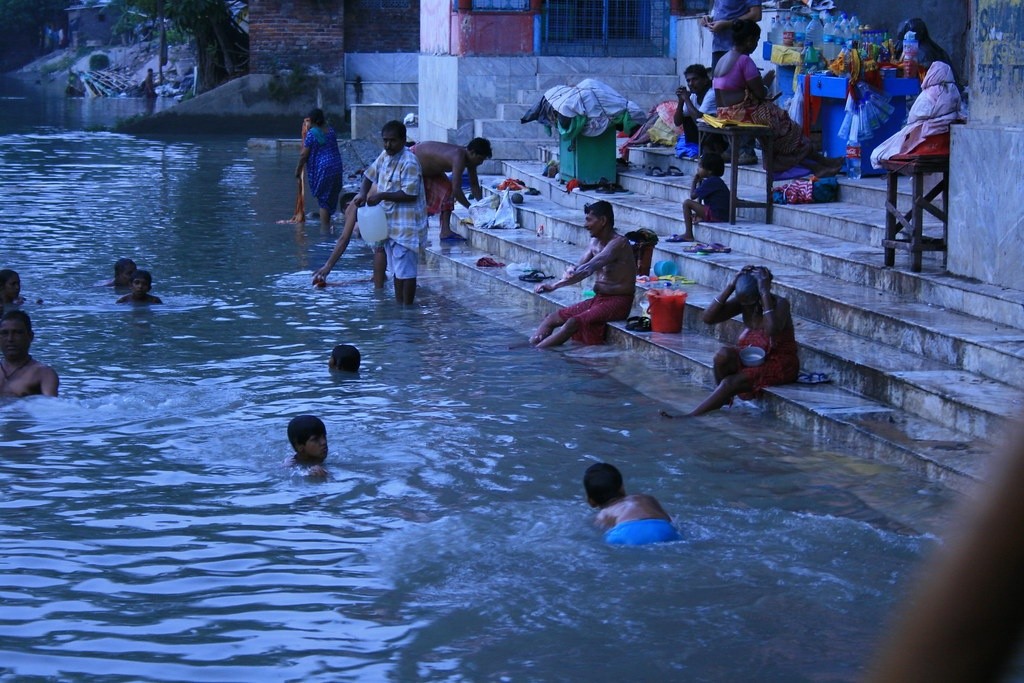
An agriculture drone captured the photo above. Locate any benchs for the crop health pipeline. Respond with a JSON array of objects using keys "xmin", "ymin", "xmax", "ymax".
[
  {"xmin": 697, "ymin": 120, "xmax": 773, "ymax": 224},
  {"xmin": 883, "ymin": 155, "xmax": 950, "ymax": 274}
]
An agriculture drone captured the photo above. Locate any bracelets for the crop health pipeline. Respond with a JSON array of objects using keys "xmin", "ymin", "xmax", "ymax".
[
  {"xmin": 714, "ymin": 298, "xmax": 724, "ymax": 306},
  {"xmin": 763, "ymin": 309, "xmax": 774, "ymax": 316}
]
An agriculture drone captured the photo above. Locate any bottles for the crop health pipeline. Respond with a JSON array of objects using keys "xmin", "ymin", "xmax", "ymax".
[
  {"xmin": 845, "ymin": 138, "xmax": 862, "ymax": 181},
  {"xmin": 838, "ymin": 79, "xmax": 895, "ymax": 141},
  {"xmin": 644, "ymin": 280, "xmax": 674, "ymax": 288},
  {"xmin": 768, "ymin": 7, "xmax": 901, "ymax": 77},
  {"xmin": 902, "ymin": 32, "xmax": 918, "ymax": 77}
]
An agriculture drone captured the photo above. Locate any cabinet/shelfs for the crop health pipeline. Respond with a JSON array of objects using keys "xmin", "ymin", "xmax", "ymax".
[
  {"xmin": 559, "ymin": 126, "xmax": 617, "ymax": 189},
  {"xmin": 796, "ymin": 74, "xmax": 920, "ymax": 175}
]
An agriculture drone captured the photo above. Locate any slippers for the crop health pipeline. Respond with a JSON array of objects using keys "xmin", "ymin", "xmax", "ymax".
[
  {"xmin": 645, "ymin": 166, "xmax": 665, "ymax": 177},
  {"xmin": 625, "ymin": 316, "xmax": 651, "ymax": 331},
  {"xmin": 519, "ymin": 271, "xmax": 555, "ymax": 282},
  {"xmin": 665, "ymin": 234, "xmax": 688, "ymax": 241},
  {"xmin": 683, "ymin": 243, "xmax": 713, "ymax": 252},
  {"xmin": 797, "ymin": 372, "xmax": 830, "ymax": 384},
  {"xmin": 441, "ymin": 234, "xmax": 467, "ymax": 241},
  {"xmin": 664, "ymin": 166, "xmax": 684, "ymax": 175},
  {"xmin": 596, "ymin": 183, "xmax": 629, "ymax": 193},
  {"xmin": 709, "ymin": 242, "xmax": 731, "ymax": 253}
]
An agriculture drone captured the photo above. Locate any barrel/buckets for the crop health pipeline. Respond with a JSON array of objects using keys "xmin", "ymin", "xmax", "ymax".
[
  {"xmin": 654, "ymin": 260, "xmax": 681, "ymax": 278},
  {"xmin": 357, "ymin": 200, "xmax": 389, "ymax": 243},
  {"xmin": 646, "ymin": 289, "xmax": 687, "ymax": 334}
]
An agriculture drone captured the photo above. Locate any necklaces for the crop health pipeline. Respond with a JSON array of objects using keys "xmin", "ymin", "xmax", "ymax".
[{"xmin": 0, "ymin": 354, "xmax": 32, "ymax": 383}]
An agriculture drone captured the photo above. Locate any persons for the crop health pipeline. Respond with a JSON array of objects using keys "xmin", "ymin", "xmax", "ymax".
[
  {"xmin": 145, "ymin": 68, "xmax": 158, "ymax": 98},
  {"xmin": 102, "ymin": 258, "xmax": 163, "ymax": 304},
  {"xmin": 659, "ymin": 264, "xmax": 800, "ymax": 419},
  {"xmin": 0, "ymin": 269, "xmax": 24, "ymax": 315},
  {"xmin": 583, "ymin": 463, "xmax": 679, "ymax": 545},
  {"xmin": 674, "ymin": 64, "xmax": 728, "ymax": 160},
  {"xmin": 281, "ymin": 415, "xmax": 329, "ymax": 479},
  {"xmin": 865, "ymin": 412, "xmax": 1024, "ymax": 683},
  {"xmin": 295, "ymin": 108, "xmax": 344, "ymax": 226},
  {"xmin": 329, "ymin": 345, "xmax": 361, "ymax": 382},
  {"xmin": 700, "ymin": 0, "xmax": 762, "ymax": 79},
  {"xmin": 896, "ymin": 17, "xmax": 964, "ymax": 93},
  {"xmin": 354, "ymin": 77, "xmax": 364, "ymax": 104},
  {"xmin": 312, "ymin": 192, "xmax": 386, "ymax": 287},
  {"xmin": 528, "ymin": 200, "xmax": 638, "ymax": 349},
  {"xmin": 408, "ymin": 138, "xmax": 492, "ymax": 239},
  {"xmin": 354, "ymin": 119, "xmax": 428, "ymax": 305},
  {"xmin": 672, "ymin": 153, "xmax": 731, "ymax": 241},
  {"xmin": 0, "ymin": 310, "xmax": 59, "ymax": 399},
  {"xmin": 713, "ymin": 19, "xmax": 845, "ymax": 177}
]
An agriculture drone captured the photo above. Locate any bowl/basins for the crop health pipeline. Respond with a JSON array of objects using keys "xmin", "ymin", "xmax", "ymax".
[{"xmin": 740, "ymin": 346, "xmax": 766, "ymax": 365}]
